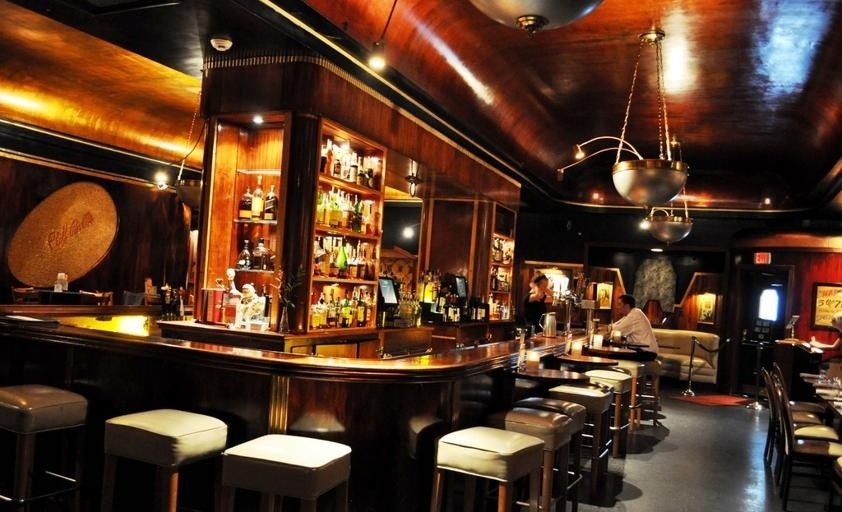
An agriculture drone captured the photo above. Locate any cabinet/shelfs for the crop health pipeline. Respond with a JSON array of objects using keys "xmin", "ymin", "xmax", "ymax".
[
  {"xmin": 200, "ymin": 113, "xmax": 384, "ymax": 332},
  {"xmin": 290, "ymin": 338, "xmax": 379, "ymax": 359},
  {"xmin": 488, "ymin": 232, "xmax": 514, "ymax": 320}
]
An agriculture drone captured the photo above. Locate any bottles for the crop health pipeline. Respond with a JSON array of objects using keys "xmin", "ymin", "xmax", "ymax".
[
  {"xmin": 310, "ymin": 135, "xmax": 377, "ymax": 328},
  {"xmin": 55, "ymin": 273, "xmax": 68, "ymax": 290},
  {"xmin": 490, "ymin": 237, "xmax": 512, "ymax": 319},
  {"xmin": 393, "ymin": 281, "xmax": 421, "ymax": 326},
  {"xmin": 201, "ymin": 174, "xmax": 277, "ymax": 334},
  {"xmin": 159, "ymin": 284, "xmax": 185, "ymax": 321},
  {"xmin": 422, "ymin": 268, "xmax": 489, "ymax": 323}
]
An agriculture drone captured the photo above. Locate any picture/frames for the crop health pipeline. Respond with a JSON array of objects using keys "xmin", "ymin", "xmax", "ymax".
[
  {"xmin": 696, "ymin": 274, "xmax": 718, "ymax": 325},
  {"xmin": 808, "ymin": 282, "xmax": 842, "ymax": 331},
  {"xmin": 594, "ymin": 269, "xmax": 616, "ymax": 311}
]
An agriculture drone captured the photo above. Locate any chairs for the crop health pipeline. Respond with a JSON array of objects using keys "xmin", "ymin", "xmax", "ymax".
[{"xmin": 760, "ymin": 361, "xmax": 842, "ymax": 512}]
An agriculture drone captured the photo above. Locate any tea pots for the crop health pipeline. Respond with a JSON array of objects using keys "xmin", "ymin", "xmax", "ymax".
[{"xmin": 538, "ymin": 311, "xmax": 559, "ymax": 338}]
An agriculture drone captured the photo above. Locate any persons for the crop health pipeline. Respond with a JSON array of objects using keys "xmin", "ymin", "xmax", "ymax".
[
  {"xmin": 522, "ymin": 273, "xmax": 555, "ymax": 334},
  {"xmin": 600, "ymin": 295, "xmax": 659, "ymax": 364},
  {"xmin": 809, "ymin": 312, "xmax": 842, "ymax": 377}
]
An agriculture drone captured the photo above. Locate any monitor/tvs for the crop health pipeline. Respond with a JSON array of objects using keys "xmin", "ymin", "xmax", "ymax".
[{"xmin": 378, "ymin": 277, "xmax": 399, "ymax": 305}]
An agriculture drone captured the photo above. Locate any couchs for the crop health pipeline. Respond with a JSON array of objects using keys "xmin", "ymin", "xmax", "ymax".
[{"xmin": 652, "ymin": 328, "xmax": 721, "ymax": 384}]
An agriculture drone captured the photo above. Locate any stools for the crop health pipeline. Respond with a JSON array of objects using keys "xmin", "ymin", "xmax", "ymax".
[
  {"xmin": 428, "ymin": 358, "xmax": 663, "ymax": 512},
  {"xmin": 100, "ymin": 408, "xmax": 228, "ymax": 512},
  {"xmin": 217, "ymin": 433, "xmax": 353, "ymax": 512},
  {"xmin": 0, "ymin": 385, "xmax": 89, "ymax": 512}
]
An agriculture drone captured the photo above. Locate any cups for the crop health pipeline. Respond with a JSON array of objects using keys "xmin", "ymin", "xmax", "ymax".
[
  {"xmin": 559, "ymin": 298, "xmax": 572, "ymax": 336},
  {"xmin": 594, "ymin": 335, "xmax": 604, "ymax": 348},
  {"xmin": 514, "ymin": 326, "xmax": 535, "ymax": 340},
  {"xmin": 526, "ymin": 351, "xmax": 540, "ymax": 373},
  {"xmin": 54, "ymin": 284, "xmax": 62, "ymax": 292},
  {"xmin": 584, "ymin": 318, "xmax": 601, "ymax": 334},
  {"xmin": 571, "ymin": 342, "xmax": 583, "ymax": 358},
  {"xmin": 614, "ymin": 331, "xmax": 622, "ymax": 343},
  {"xmin": 378, "ymin": 311, "xmax": 386, "ymax": 328}
]
